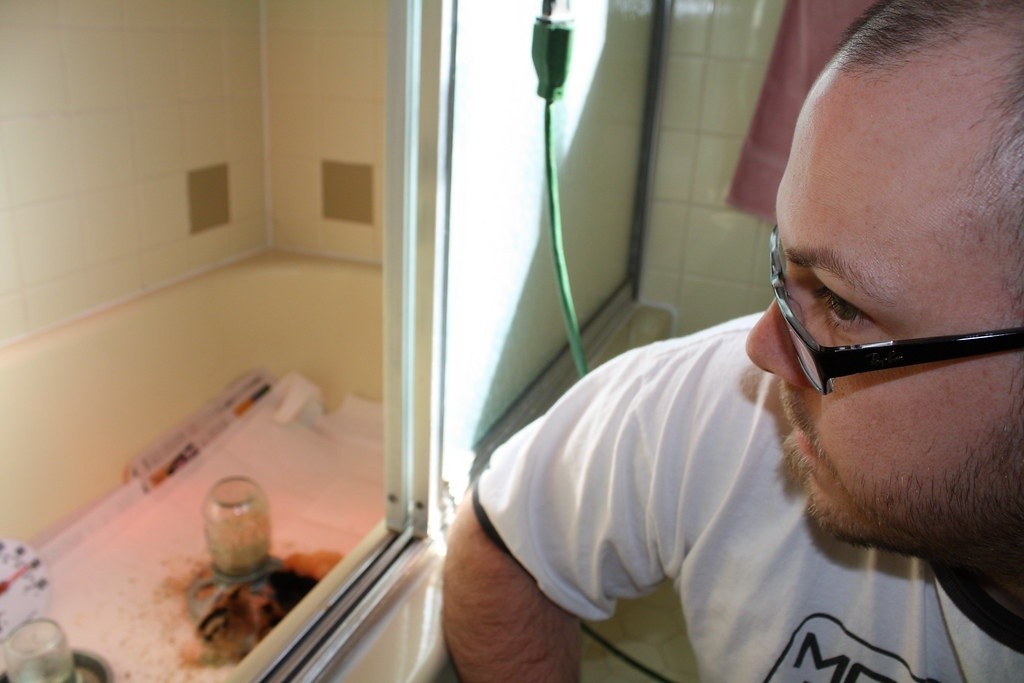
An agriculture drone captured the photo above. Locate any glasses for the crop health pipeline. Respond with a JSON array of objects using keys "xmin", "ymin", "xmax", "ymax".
[{"xmin": 769, "ymin": 225, "xmax": 1023, "ymax": 396}]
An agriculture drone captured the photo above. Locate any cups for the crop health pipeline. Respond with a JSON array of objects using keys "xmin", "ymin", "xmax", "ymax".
[
  {"xmin": 203, "ymin": 475, "xmax": 272, "ymax": 579},
  {"xmin": 4, "ymin": 618, "xmax": 83, "ymax": 682}
]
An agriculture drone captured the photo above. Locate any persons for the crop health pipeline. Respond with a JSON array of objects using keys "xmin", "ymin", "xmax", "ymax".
[{"xmin": 442, "ymin": 0, "xmax": 1024, "ymax": 683}]
